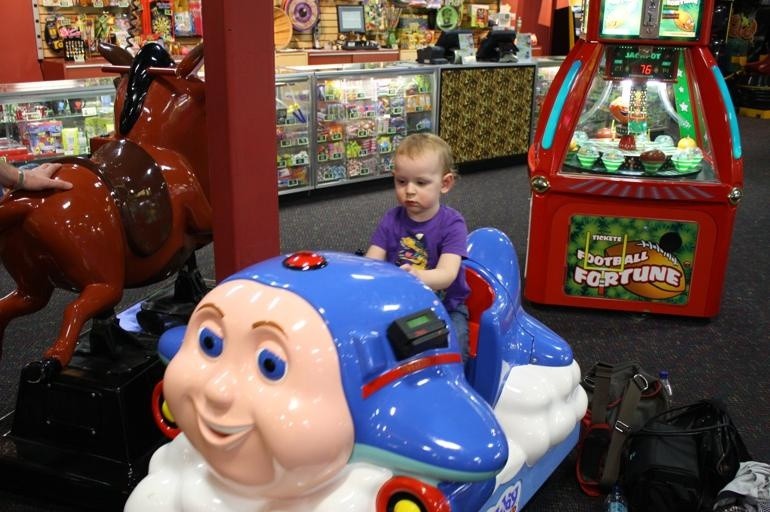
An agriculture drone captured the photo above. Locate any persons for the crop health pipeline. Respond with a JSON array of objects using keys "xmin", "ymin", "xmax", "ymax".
[
  {"xmin": 361, "ymin": 134, "xmax": 468, "ymax": 368},
  {"xmin": 1, "ymin": 157, "xmax": 74, "ymax": 193}
]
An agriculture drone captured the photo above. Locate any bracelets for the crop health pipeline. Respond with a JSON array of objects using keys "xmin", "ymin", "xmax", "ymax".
[{"xmin": 9, "ymin": 169, "xmax": 24, "ymax": 194}]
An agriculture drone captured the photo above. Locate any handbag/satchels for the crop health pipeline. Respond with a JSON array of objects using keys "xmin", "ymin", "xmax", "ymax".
[{"xmin": 567, "ymin": 362, "xmax": 770, "ymax": 512}]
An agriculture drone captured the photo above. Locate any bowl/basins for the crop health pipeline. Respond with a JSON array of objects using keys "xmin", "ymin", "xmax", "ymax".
[{"xmin": 570, "ymin": 133, "xmax": 706, "ymax": 174}]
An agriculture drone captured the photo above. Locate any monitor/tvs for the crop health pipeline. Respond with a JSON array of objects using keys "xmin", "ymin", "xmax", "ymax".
[
  {"xmin": 337, "ymin": 4, "xmax": 366, "ymax": 34},
  {"xmin": 599, "ymin": 0, "xmax": 643, "ymax": 39},
  {"xmin": 659, "ymin": 0, "xmax": 705, "ymax": 41},
  {"xmin": 437, "ymin": 31, "xmax": 474, "ymax": 64},
  {"xmin": 476, "ymin": 30, "xmax": 519, "ymax": 62}
]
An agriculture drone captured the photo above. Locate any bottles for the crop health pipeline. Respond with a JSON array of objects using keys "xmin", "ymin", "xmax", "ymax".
[{"xmin": 656, "ymin": 371, "xmax": 674, "ymax": 416}]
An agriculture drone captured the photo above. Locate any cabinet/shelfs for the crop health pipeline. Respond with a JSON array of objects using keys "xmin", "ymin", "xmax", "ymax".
[{"xmin": 0, "ymin": 75, "xmax": 119, "ymax": 203}]
